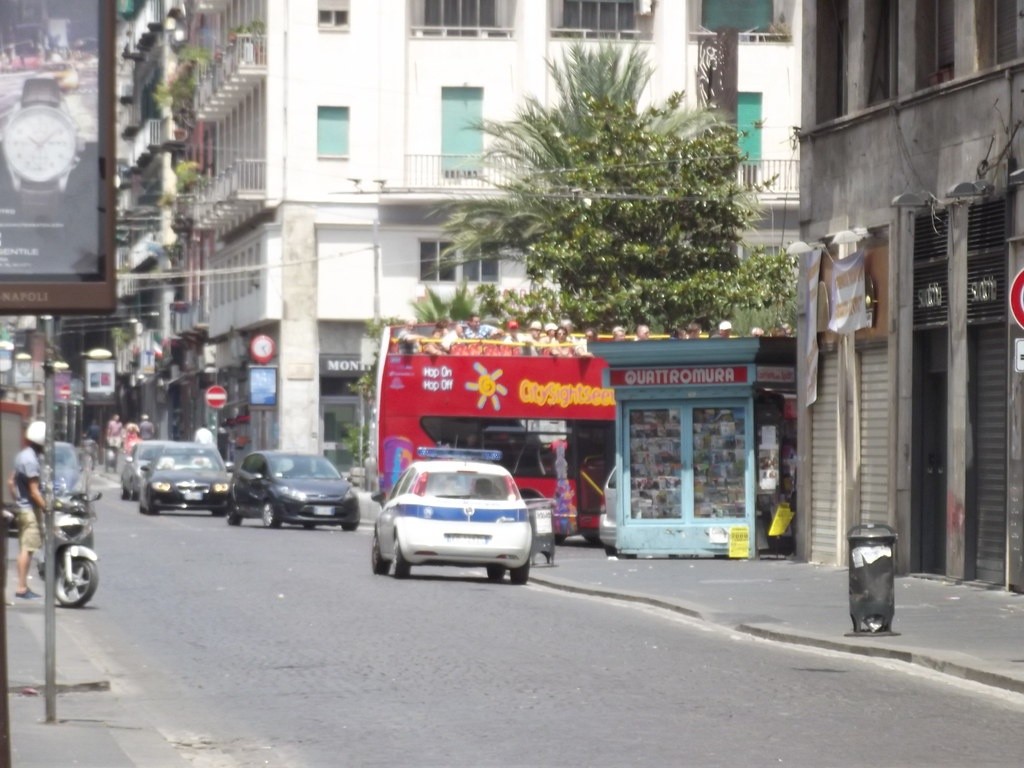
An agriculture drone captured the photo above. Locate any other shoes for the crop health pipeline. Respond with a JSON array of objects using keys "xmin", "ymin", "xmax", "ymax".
[{"xmin": 17, "ymin": 586, "xmax": 44, "ymax": 602}]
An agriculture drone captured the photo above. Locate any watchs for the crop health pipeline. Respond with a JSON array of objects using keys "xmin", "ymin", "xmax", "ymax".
[{"xmin": 2, "ymin": 79, "xmax": 82, "ymax": 222}]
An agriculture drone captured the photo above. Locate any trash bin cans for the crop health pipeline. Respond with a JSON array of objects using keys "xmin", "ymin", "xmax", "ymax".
[
  {"xmin": 843, "ymin": 523, "xmax": 903, "ymax": 637},
  {"xmin": 522, "ymin": 498, "xmax": 559, "ymax": 569}
]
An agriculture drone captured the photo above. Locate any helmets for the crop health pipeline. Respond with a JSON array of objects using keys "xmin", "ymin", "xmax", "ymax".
[{"xmin": 26, "ymin": 419, "xmax": 47, "ymax": 446}]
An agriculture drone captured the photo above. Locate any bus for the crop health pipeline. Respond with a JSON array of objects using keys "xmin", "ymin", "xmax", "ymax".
[{"xmin": 375, "ymin": 321, "xmax": 615, "ymax": 543}]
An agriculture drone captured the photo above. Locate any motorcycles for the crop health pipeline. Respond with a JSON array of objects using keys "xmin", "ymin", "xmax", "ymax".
[{"xmin": 38, "ymin": 482, "xmax": 100, "ymax": 606}]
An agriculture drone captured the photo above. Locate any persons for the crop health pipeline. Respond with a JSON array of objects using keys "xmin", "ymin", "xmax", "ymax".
[
  {"xmin": 82, "ymin": 418, "xmax": 100, "ymax": 443},
  {"xmin": 194, "ymin": 423, "xmax": 212, "ymax": 444},
  {"xmin": 6, "ymin": 419, "xmax": 53, "ymax": 598},
  {"xmin": 137, "ymin": 414, "xmax": 155, "ymax": 440},
  {"xmin": 106, "ymin": 414, "xmax": 123, "ymax": 455},
  {"xmin": 217, "ymin": 421, "xmax": 232, "ymax": 461},
  {"xmin": 122, "ymin": 422, "xmax": 140, "ymax": 456}
]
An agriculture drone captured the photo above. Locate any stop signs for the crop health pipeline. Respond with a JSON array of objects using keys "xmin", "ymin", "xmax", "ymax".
[{"xmin": 206, "ymin": 385, "xmax": 227, "ymax": 408}]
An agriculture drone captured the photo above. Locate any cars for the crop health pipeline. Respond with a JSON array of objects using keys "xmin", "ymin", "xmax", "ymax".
[
  {"xmin": 372, "ymin": 447, "xmax": 533, "ymax": 584},
  {"xmin": 227, "ymin": 451, "xmax": 360, "ymax": 531},
  {"xmin": 122, "ymin": 441, "xmax": 166, "ymax": 501},
  {"xmin": 141, "ymin": 442, "xmax": 234, "ymax": 517},
  {"xmin": 599, "ymin": 465, "xmax": 616, "ymax": 556},
  {"xmin": 53, "ymin": 442, "xmax": 86, "ymax": 493}
]
